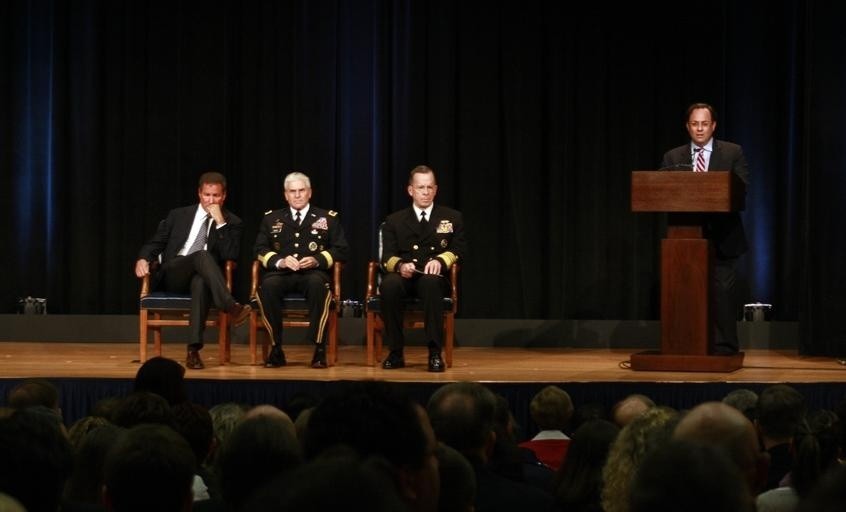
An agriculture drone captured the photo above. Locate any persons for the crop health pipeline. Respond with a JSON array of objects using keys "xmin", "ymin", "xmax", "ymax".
[
  {"xmin": 659, "ymin": 101, "xmax": 749, "ymax": 354},
  {"xmin": 378, "ymin": 165, "xmax": 469, "ymax": 368},
  {"xmin": 134, "ymin": 172, "xmax": 251, "ymax": 369},
  {"xmin": 1, "ymin": 356, "xmax": 846, "ymax": 511},
  {"xmin": 252, "ymin": 171, "xmax": 349, "ymax": 369}
]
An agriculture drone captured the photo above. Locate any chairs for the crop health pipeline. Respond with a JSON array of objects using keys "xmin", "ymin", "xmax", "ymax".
[
  {"xmin": 136, "ymin": 217, "xmax": 238, "ymax": 367},
  {"xmin": 248, "ymin": 251, "xmax": 343, "ymax": 368},
  {"xmin": 364, "ymin": 221, "xmax": 457, "ymax": 368}
]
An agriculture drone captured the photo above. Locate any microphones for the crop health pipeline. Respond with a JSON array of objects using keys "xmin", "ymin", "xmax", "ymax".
[{"xmin": 693, "ymin": 147, "xmax": 701, "ymax": 152}]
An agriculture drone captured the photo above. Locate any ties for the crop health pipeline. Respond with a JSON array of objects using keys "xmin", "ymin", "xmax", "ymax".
[
  {"xmin": 420, "ymin": 210, "xmax": 427, "ymax": 227},
  {"xmin": 295, "ymin": 212, "xmax": 301, "ymax": 225},
  {"xmin": 187, "ymin": 215, "xmax": 212, "ymax": 255},
  {"xmin": 696, "ymin": 147, "xmax": 705, "ymax": 172}
]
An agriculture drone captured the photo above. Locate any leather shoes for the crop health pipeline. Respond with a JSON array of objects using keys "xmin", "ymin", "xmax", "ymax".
[
  {"xmin": 429, "ymin": 350, "xmax": 444, "ymax": 372},
  {"xmin": 265, "ymin": 349, "xmax": 287, "ymax": 367},
  {"xmin": 186, "ymin": 349, "xmax": 204, "ymax": 369},
  {"xmin": 383, "ymin": 352, "xmax": 404, "ymax": 369},
  {"xmin": 229, "ymin": 301, "xmax": 251, "ymax": 329},
  {"xmin": 311, "ymin": 349, "xmax": 328, "ymax": 368}
]
